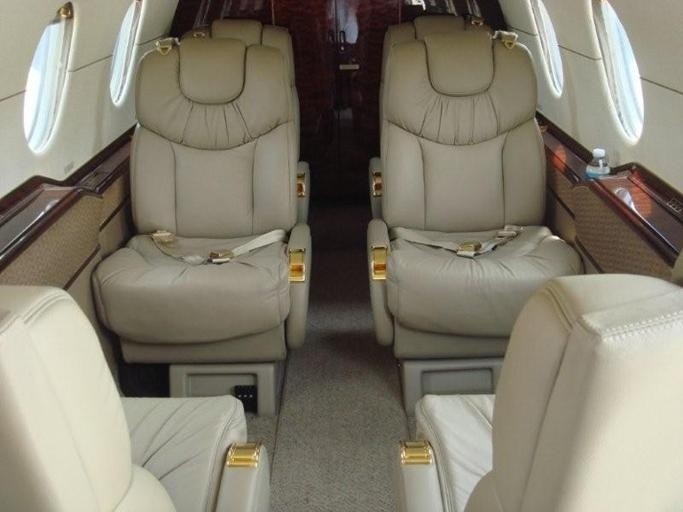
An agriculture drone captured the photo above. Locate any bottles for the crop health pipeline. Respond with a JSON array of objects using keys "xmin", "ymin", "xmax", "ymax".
[{"xmin": 585, "ymin": 148, "xmax": 611, "ymax": 182}]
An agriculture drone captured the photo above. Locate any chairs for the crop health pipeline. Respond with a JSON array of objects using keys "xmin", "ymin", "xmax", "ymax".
[
  {"xmin": 183, "ymin": 20, "xmax": 311, "ymax": 221},
  {"xmin": 400, "ymin": 268, "xmax": 683, "ymax": 510},
  {"xmin": 368, "ymin": 16, "xmax": 489, "ymax": 222},
  {"xmin": 0, "ymin": 280, "xmax": 273, "ymax": 512},
  {"xmin": 92, "ymin": 37, "xmax": 312, "ymax": 367},
  {"xmin": 367, "ymin": 30, "xmax": 586, "ymax": 361}
]
